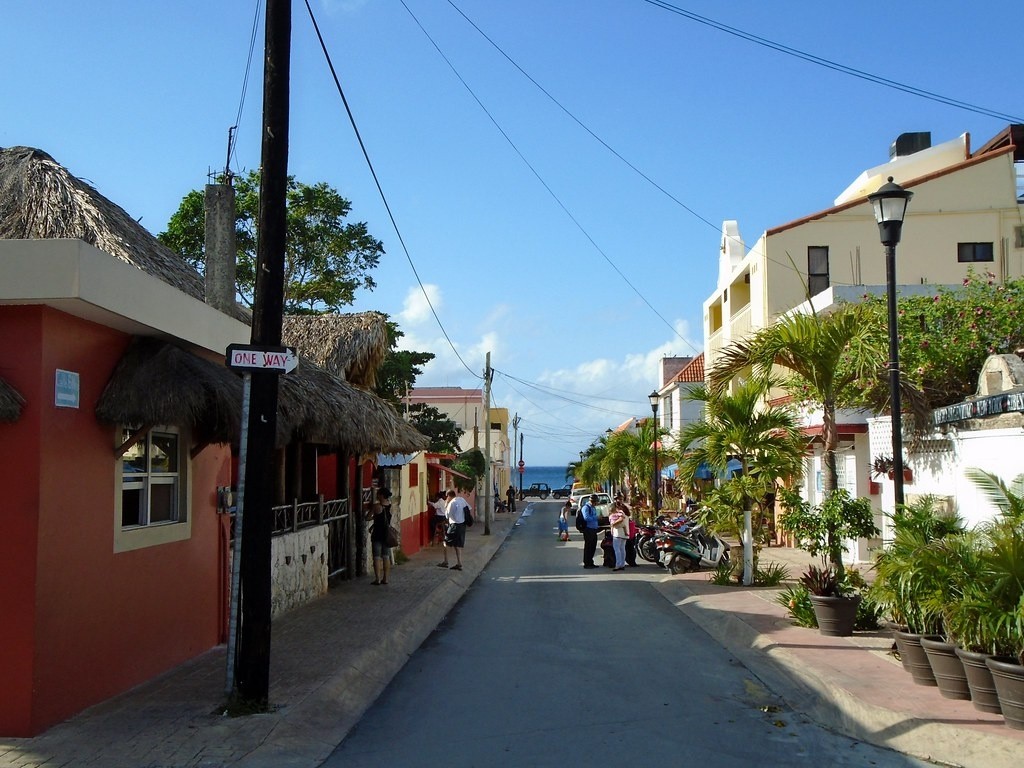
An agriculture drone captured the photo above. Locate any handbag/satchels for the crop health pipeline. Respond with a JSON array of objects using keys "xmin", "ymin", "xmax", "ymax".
[
  {"xmin": 385, "ymin": 524, "xmax": 399, "ymax": 548},
  {"xmin": 459, "ymin": 497, "xmax": 475, "ymax": 527}
]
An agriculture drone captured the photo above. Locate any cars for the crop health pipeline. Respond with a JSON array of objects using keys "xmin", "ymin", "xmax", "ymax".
[
  {"xmin": 576, "ymin": 492, "xmax": 614, "ymax": 526},
  {"xmin": 551, "ymin": 484, "xmax": 572, "ymax": 499},
  {"xmin": 569, "ymin": 488, "xmax": 592, "ymax": 516}
]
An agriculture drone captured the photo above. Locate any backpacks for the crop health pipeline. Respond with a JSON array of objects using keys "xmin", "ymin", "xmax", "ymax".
[{"xmin": 576, "ymin": 504, "xmax": 591, "ymax": 534}]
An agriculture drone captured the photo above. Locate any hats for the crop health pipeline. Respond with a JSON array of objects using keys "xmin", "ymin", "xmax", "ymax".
[
  {"xmin": 588, "ymin": 494, "xmax": 599, "ymax": 501},
  {"xmin": 614, "ymin": 491, "xmax": 625, "ymax": 500}
]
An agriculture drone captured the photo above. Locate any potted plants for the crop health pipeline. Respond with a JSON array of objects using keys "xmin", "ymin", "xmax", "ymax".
[
  {"xmin": 778, "ymin": 484, "xmax": 882, "ymax": 637},
  {"xmin": 689, "ymin": 470, "xmax": 776, "ymax": 579},
  {"xmin": 867, "ymin": 468, "xmax": 1024, "ymax": 730}
]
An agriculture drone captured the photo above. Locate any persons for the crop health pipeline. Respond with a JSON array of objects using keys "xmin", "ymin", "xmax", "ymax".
[
  {"xmin": 494, "ymin": 483, "xmax": 519, "ymax": 514},
  {"xmin": 366, "ymin": 488, "xmax": 392, "ymax": 586},
  {"xmin": 557, "ymin": 483, "xmax": 771, "ymax": 572},
  {"xmin": 427, "ymin": 489, "xmax": 472, "ymax": 571}
]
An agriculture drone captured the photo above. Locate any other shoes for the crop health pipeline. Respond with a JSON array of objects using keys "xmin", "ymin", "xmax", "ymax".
[
  {"xmin": 583, "ymin": 564, "xmax": 599, "ymax": 570},
  {"xmin": 438, "ymin": 561, "xmax": 449, "ymax": 567},
  {"xmin": 381, "ymin": 579, "xmax": 389, "ymax": 584},
  {"xmin": 450, "ymin": 564, "xmax": 463, "ymax": 571},
  {"xmin": 613, "ymin": 567, "xmax": 625, "ymax": 571},
  {"xmin": 370, "ymin": 580, "xmax": 381, "ymax": 585}
]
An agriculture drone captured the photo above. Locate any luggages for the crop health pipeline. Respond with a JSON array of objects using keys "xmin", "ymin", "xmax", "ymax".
[{"xmin": 601, "ymin": 527, "xmax": 617, "ymax": 569}]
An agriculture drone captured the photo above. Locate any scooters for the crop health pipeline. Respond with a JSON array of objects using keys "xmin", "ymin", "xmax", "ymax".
[{"xmin": 632, "ymin": 502, "xmax": 734, "ymax": 576}]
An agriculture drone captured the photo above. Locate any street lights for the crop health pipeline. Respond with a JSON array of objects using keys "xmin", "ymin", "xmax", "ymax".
[
  {"xmin": 578, "ymin": 450, "xmax": 585, "ymax": 463},
  {"xmin": 866, "ymin": 175, "xmax": 916, "ymax": 536},
  {"xmin": 648, "ymin": 390, "xmax": 662, "ymax": 519},
  {"xmin": 605, "ymin": 428, "xmax": 614, "ymax": 495}
]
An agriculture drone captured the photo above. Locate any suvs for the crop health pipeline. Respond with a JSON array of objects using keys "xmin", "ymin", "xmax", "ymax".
[{"xmin": 518, "ymin": 482, "xmax": 551, "ymax": 501}]
